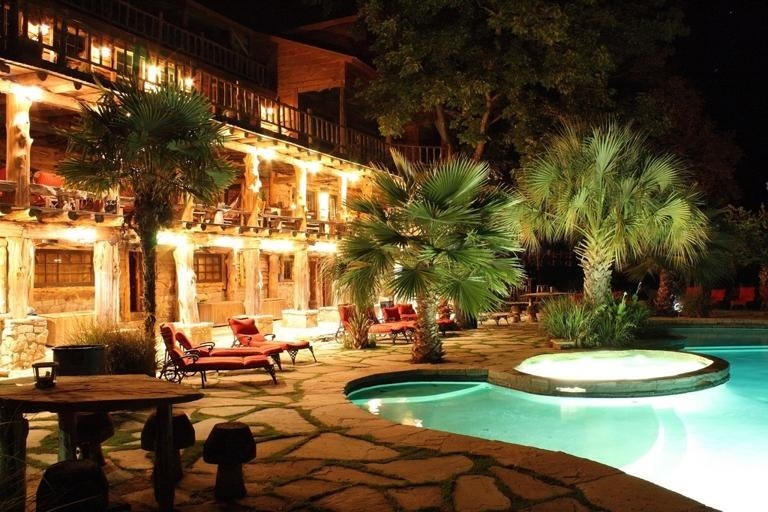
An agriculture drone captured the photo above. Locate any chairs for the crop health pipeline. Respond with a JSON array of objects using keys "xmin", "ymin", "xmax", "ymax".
[
  {"xmin": 571, "ymin": 286, "xmax": 758, "ymax": 312},
  {"xmin": 333, "ymin": 294, "xmax": 461, "ymax": 345},
  {"xmin": 154, "ymin": 322, "xmax": 282, "ymax": 391},
  {"xmin": 171, "ymin": 326, "xmax": 279, "ymax": 385},
  {"xmin": 225, "ymin": 315, "xmax": 319, "ymax": 371}
]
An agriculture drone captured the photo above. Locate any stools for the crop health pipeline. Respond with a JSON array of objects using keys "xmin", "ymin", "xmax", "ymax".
[
  {"xmin": 58, "ymin": 414, "xmax": 115, "ymax": 468},
  {"xmin": 199, "ymin": 421, "xmax": 258, "ymax": 504},
  {"xmin": 136, "ymin": 410, "xmax": 200, "ymax": 487},
  {"xmin": 33, "ymin": 458, "xmax": 113, "ymax": 512}
]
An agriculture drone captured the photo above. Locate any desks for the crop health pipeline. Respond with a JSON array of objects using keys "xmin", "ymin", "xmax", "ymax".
[
  {"xmin": 1, "ymin": 372, "xmax": 208, "ymax": 511},
  {"xmin": 482, "ymin": 289, "xmax": 570, "ymax": 327}
]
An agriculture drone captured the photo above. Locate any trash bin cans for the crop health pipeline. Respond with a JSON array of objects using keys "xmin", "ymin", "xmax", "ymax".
[{"xmin": 52, "ymin": 344, "xmax": 109, "ymax": 431}]
